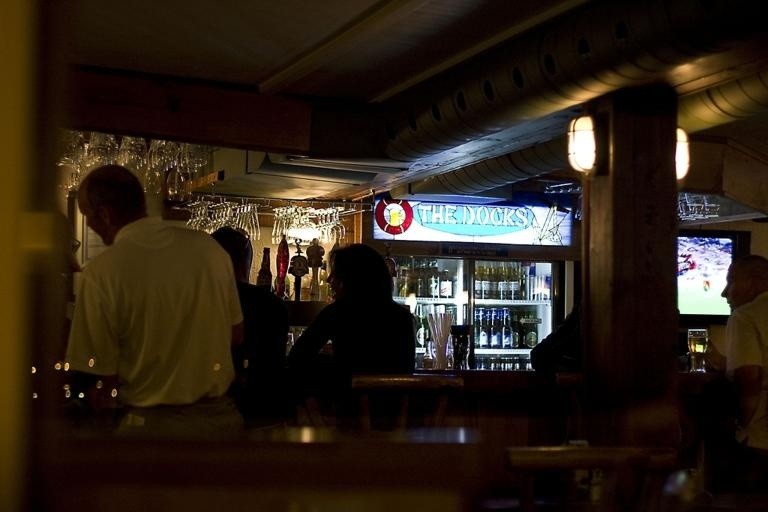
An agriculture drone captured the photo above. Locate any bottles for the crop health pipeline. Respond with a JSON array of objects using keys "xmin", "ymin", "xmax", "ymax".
[
  {"xmin": 256, "ymin": 248, "xmax": 272, "ymax": 295},
  {"xmin": 394, "ymin": 262, "xmax": 536, "ymax": 372}
]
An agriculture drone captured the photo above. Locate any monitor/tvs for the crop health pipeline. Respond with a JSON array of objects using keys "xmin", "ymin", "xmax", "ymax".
[{"xmin": 673, "ymin": 228, "xmax": 751, "ymax": 324}]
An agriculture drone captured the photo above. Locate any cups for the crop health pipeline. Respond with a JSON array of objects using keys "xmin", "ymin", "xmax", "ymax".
[{"xmin": 686, "ymin": 326, "xmax": 708, "ymax": 374}]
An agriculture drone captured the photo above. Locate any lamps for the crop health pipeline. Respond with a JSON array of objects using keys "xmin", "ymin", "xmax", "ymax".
[{"xmin": 565, "ymin": 110, "xmax": 609, "ymax": 178}]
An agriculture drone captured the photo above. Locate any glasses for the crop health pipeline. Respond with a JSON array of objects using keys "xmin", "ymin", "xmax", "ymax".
[{"xmin": 234, "ymin": 228, "xmax": 251, "ymax": 250}]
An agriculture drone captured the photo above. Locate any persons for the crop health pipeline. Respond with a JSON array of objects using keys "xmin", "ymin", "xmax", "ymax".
[
  {"xmin": 64, "ymin": 167, "xmax": 243, "ymax": 409},
  {"xmin": 276, "ymin": 245, "xmax": 415, "ymax": 418},
  {"xmin": 707, "ymin": 255, "xmax": 768, "ymax": 511},
  {"xmin": 206, "ymin": 227, "xmax": 289, "ymax": 412}
]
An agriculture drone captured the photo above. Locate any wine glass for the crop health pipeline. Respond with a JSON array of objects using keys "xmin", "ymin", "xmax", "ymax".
[{"xmin": 61, "ymin": 132, "xmax": 347, "ymax": 245}]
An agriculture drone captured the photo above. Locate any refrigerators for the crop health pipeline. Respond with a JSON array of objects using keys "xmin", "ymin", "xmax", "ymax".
[{"xmin": 371, "ymin": 197, "xmax": 582, "ymax": 382}]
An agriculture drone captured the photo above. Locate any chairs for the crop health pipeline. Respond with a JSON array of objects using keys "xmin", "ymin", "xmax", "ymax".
[
  {"xmin": 502, "ymin": 445, "xmax": 679, "ymax": 512},
  {"xmin": 350, "ymin": 373, "xmax": 467, "ymax": 428}
]
